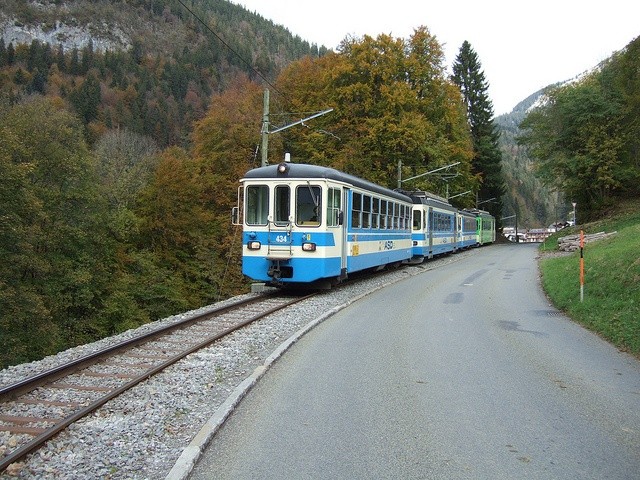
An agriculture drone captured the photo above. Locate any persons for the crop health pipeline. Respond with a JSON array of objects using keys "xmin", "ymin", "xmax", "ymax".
[{"xmin": 309, "ymin": 206, "xmax": 319, "ymax": 222}]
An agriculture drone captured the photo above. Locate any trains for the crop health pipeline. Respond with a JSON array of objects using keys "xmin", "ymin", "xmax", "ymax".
[{"xmin": 231, "ymin": 152, "xmax": 496, "ymax": 290}]
